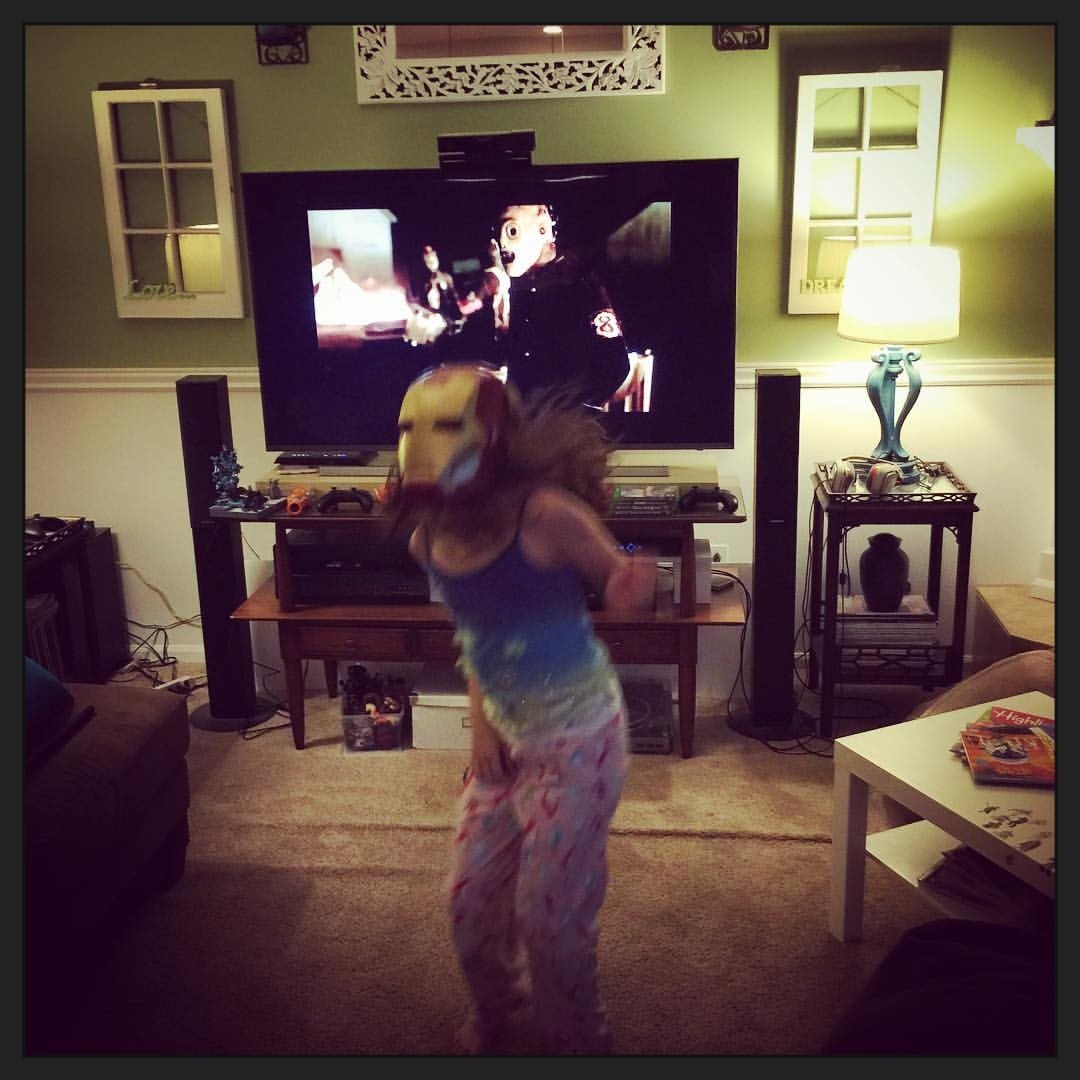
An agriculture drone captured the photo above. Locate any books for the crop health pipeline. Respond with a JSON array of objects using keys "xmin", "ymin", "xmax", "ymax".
[
  {"xmin": 949, "ymin": 707, "xmax": 1055, "ymax": 785},
  {"xmin": 836, "ymin": 595, "xmax": 939, "ymax": 664}
]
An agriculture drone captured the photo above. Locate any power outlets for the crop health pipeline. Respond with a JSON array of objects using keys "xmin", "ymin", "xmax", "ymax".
[{"xmin": 711, "ymin": 546, "xmax": 730, "ymax": 567}]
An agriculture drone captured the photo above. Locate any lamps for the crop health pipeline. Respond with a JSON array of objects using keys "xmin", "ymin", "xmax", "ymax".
[
  {"xmin": 162, "ymin": 223, "xmax": 226, "ymax": 292},
  {"xmin": 836, "ymin": 240, "xmax": 961, "ymax": 483}
]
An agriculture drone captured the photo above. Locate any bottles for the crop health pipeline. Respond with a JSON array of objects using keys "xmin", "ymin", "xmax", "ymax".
[{"xmin": 860, "ymin": 533, "xmax": 910, "ymax": 614}]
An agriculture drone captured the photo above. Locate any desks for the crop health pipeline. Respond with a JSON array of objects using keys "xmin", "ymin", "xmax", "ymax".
[
  {"xmin": 809, "ymin": 461, "xmax": 982, "ymax": 740},
  {"xmin": 200, "ymin": 462, "xmax": 747, "ymax": 616},
  {"xmin": 228, "ymin": 567, "xmax": 747, "ymax": 761}
]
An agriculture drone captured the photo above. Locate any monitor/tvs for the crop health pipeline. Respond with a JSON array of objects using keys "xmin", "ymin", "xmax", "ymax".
[{"xmin": 240, "ymin": 157, "xmax": 735, "ymax": 453}]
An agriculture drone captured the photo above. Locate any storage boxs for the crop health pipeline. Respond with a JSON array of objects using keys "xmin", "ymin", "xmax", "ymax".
[
  {"xmin": 407, "ymin": 662, "xmax": 477, "ymax": 750},
  {"xmin": 336, "ymin": 674, "xmax": 406, "ymax": 750}
]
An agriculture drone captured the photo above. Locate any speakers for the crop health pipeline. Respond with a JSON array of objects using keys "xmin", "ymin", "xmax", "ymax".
[
  {"xmin": 726, "ymin": 368, "xmax": 815, "ymax": 739},
  {"xmin": 176, "ymin": 374, "xmax": 276, "ymax": 733}
]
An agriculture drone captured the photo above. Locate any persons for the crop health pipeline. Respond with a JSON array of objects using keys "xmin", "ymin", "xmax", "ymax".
[
  {"xmin": 397, "ymin": 366, "xmax": 659, "ymax": 1040},
  {"xmin": 481, "ymin": 202, "xmax": 630, "ymax": 412}
]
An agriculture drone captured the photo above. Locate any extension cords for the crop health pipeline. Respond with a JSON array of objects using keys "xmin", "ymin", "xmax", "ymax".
[{"xmin": 155, "ymin": 675, "xmax": 196, "ymax": 693}]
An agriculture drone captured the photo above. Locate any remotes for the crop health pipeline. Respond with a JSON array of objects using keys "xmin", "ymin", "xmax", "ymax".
[{"xmin": 272, "ymin": 449, "xmax": 367, "ymax": 467}]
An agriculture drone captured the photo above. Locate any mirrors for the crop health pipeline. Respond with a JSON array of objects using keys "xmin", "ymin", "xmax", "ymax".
[{"xmin": 350, "ymin": 24, "xmax": 666, "ymax": 105}]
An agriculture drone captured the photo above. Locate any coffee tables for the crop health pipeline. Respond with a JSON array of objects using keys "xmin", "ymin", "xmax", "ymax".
[{"xmin": 827, "ymin": 690, "xmax": 1058, "ymax": 946}]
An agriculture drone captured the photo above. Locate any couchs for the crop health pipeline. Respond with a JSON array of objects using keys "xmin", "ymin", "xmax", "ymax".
[{"xmin": 19, "ymin": 680, "xmax": 192, "ymax": 1024}]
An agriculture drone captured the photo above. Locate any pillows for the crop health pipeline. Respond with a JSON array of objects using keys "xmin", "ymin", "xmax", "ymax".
[{"xmin": 23, "ymin": 634, "xmax": 95, "ymax": 772}]
